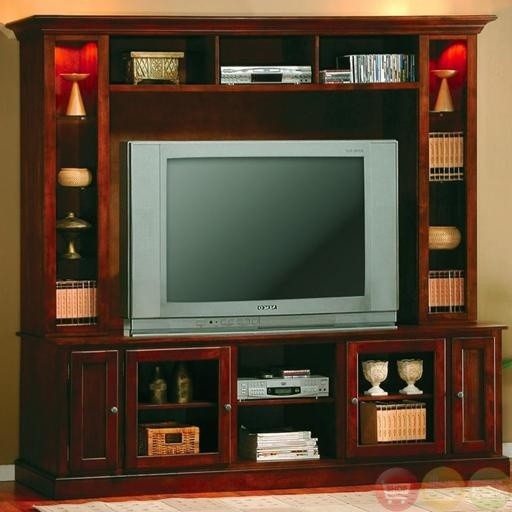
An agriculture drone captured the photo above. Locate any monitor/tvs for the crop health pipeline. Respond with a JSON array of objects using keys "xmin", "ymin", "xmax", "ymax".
[{"xmin": 120, "ymin": 139, "xmax": 402, "ymax": 337}]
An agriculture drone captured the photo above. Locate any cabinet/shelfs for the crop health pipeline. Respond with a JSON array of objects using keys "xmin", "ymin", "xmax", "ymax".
[
  {"xmin": 14, "ymin": 320, "xmax": 512, "ymax": 504},
  {"xmin": 3, "ymin": 13, "xmax": 498, "ymax": 336}
]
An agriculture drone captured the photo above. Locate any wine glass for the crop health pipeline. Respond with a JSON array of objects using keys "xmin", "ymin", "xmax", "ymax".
[
  {"xmin": 396, "ymin": 359, "xmax": 424, "ymax": 396},
  {"xmin": 362, "ymin": 360, "xmax": 388, "ymax": 396}
]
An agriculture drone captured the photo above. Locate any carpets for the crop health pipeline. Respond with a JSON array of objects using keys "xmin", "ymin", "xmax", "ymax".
[{"xmin": 31, "ymin": 483, "xmax": 511, "ymax": 512}]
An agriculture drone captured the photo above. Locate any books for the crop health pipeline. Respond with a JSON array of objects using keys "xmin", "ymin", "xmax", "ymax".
[{"xmin": 237, "ymin": 426, "xmax": 321, "ymax": 462}]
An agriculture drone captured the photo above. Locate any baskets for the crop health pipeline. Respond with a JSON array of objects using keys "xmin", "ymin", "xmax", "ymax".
[{"xmin": 139, "ymin": 422, "xmax": 200, "ymax": 456}]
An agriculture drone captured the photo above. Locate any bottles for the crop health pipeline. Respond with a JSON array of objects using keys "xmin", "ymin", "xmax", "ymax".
[
  {"xmin": 147, "ymin": 362, "xmax": 169, "ymax": 405},
  {"xmin": 170, "ymin": 362, "xmax": 194, "ymax": 404}
]
